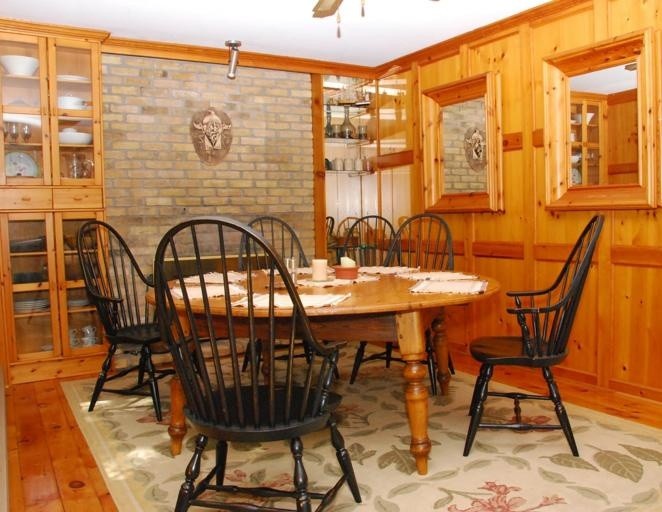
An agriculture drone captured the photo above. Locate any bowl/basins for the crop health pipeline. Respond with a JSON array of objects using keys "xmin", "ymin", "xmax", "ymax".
[
  {"xmin": 1, "ymin": 55, "xmax": 39, "ymax": 77},
  {"xmin": 60, "ymin": 133, "xmax": 92, "ymax": 146},
  {"xmin": 61, "ymin": 97, "xmax": 86, "ymax": 109},
  {"xmin": 334, "ymin": 266, "xmax": 359, "ymax": 279}
]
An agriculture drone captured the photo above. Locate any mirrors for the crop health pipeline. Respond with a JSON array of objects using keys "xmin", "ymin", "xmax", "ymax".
[
  {"xmin": 418, "ymin": 70, "xmax": 506, "ymax": 215},
  {"xmin": 539, "ymin": 24, "xmax": 656, "ymax": 212}
]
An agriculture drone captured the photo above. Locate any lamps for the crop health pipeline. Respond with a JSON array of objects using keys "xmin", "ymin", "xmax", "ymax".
[{"xmin": 224, "ymin": 41, "xmax": 242, "ymax": 79}]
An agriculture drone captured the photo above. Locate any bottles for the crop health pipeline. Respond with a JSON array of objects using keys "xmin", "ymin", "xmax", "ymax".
[
  {"xmin": 340, "ymin": 106, "xmax": 357, "ymax": 139},
  {"xmin": 325, "ymin": 106, "xmax": 334, "ymax": 137}
]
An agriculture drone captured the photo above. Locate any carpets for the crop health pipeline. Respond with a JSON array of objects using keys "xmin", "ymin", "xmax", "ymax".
[{"xmin": 59, "ymin": 335, "xmax": 661, "ymax": 512}]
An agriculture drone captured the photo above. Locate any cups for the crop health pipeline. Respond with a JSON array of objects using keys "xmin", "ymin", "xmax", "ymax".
[
  {"xmin": 69, "ymin": 324, "xmax": 99, "ymax": 347},
  {"xmin": 64, "ymin": 154, "xmax": 92, "ymax": 177},
  {"xmin": 358, "ymin": 125, "xmax": 367, "ymax": 140},
  {"xmin": 332, "ymin": 158, "xmax": 365, "ymax": 170},
  {"xmin": 331, "ymin": 124, "xmax": 341, "ymax": 139},
  {"xmin": 284, "ymin": 257, "xmax": 298, "ymax": 289},
  {"xmin": 311, "ymin": 258, "xmax": 328, "ymax": 282}
]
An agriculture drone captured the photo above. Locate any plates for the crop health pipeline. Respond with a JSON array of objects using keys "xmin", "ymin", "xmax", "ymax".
[
  {"xmin": 60, "ymin": 74, "xmax": 89, "ymax": 81},
  {"xmin": 6, "ymin": 151, "xmax": 41, "ymax": 178},
  {"xmin": 14, "ymin": 299, "xmax": 50, "ymax": 313},
  {"xmin": 68, "ymin": 298, "xmax": 92, "ymax": 309}
]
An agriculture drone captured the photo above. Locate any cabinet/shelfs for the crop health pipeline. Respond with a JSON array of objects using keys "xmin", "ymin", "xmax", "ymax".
[
  {"xmin": 324, "ymin": 101, "xmax": 375, "ymax": 175},
  {"xmin": 1, "ymin": 17, "xmax": 116, "ymax": 388},
  {"xmin": 568, "ymin": 89, "xmax": 607, "ymax": 186},
  {"xmin": 75, "ymin": 211, "xmax": 605, "ymax": 512}
]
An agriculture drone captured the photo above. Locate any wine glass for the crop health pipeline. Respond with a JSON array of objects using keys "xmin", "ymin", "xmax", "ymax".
[{"xmin": 3, "ymin": 120, "xmax": 33, "ymax": 144}]
